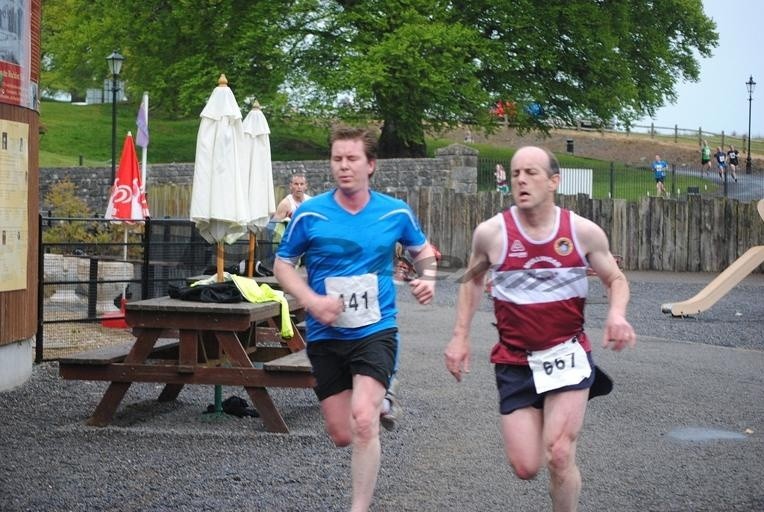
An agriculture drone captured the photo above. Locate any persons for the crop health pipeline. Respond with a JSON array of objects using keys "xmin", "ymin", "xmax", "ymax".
[
  {"xmin": 444, "ymin": 142, "xmax": 637, "ymax": 512},
  {"xmin": 272, "ymin": 173, "xmax": 313, "ymax": 220},
  {"xmin": 271, "ymin": 124, "xmax": 440, "ymax": 512},
  {"xmin": 492, "ymin": 163, "xmax": 509, "ymax": 194},
  {"xmin": 725, "ymin": 144, "xmax": 741, "ymax": 183},
  {"xmin": 651, "ymin": 154, "xmax": 671, "ymax": 199},
  {"xmin": 697, "ymin": 139, "xmax": 712, "ymax": 179},
  {"xmin": 714, "ymin": 147, "xmax": 728, "ymax": 183}
]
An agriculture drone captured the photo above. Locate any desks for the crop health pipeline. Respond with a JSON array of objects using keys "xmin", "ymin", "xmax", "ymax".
[{"xmin": 85, "ymin": 293, "xmax": 299, "ymax": 432}]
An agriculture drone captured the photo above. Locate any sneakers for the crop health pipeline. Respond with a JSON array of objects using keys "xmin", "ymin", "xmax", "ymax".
[
  {"xmin": 587, "ymin": 364, "xmax": 614, "ymax": 401},
  {"xmin": 378, "ymin": 378, "xmax": 403, "ymax": 432}
]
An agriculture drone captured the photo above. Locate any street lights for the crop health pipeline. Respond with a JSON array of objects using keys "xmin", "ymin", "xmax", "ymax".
[
  {"xmin": 103, "ymin": 49, "xmax": 125, "ymax": 190},
  {"xmin": 745, "ymin": 75, "xmax": 757, "ymax": 175}
]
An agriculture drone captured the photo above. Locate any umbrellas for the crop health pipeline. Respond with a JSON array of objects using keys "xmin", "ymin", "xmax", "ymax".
[
  {"xmin": 189, "ymin": 72, "xmax": 249, "ymax": 281},
  {"xmin": 102, "ymin": 132, "xmax": 150, "ymax": 318},
  {"xmin": 242, "ymin": 97, "xmax": 277, "ymax": 280}
]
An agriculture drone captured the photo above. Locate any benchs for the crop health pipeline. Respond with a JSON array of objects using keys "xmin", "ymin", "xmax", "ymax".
[{"xmin": 57, "ymin": 336, "xmax": 312, "ymax": 434}]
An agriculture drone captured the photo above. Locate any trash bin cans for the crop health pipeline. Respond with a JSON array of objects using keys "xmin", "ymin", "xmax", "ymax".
[{"xmin": 567, "ymin": 139, "xmax": 574, "ymax": 155}]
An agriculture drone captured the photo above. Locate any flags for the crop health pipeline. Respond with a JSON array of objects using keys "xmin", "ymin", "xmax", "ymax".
[{"xmin": 134, "ymin": 94, "xmax": 151, "ymax": 148}]
